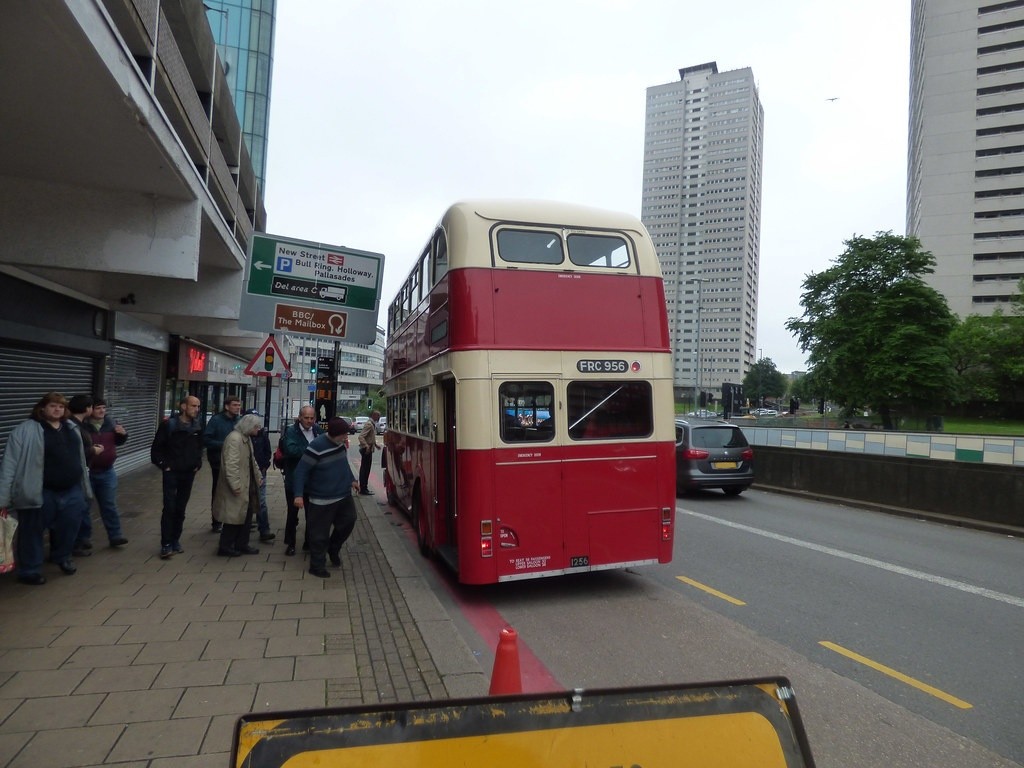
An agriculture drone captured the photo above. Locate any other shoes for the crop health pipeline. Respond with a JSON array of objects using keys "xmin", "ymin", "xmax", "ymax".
[
  {"xmin": 234, "ymin": 545, "xmax": 259, "ymax": 554},
  {"xmin": 173, "ymin": 543, "xmax": 184, "ymax": 553},
  {"xmin": 285, "ymin": 546, "xmax": 295, "ymax": 555},
  {"xmin": 217, "ymin": 547, "xmax": 240, "ymax": 557},
  {"xmin": 161, "ymin": 544, "xmax": 172, "ymax": 556},
  {"xmin": 302, "ymin": 542, "xmax": 309, "ymax": 550},
  {"xmin": 214, "ymin": 526, "xmax": 222, "ymax": 532},
  {"xmin": 260, "ymin": 533, "xmax": 275, "ymax": 540},
  {"xmin": 77, "ymin": 537, "xmax": 92, "ymax": 549},
  {"xmin": 110, "ymin": 537, "xmax": 129, "ymax": 546},
  {"xmin": 361, "ymin": 489, "xmax": 374, "ymax": 495},
  {"xmin": 50, "ymin": 552, "xmax": 77, "ymax": 575},
  {"xmin": 18, "ymin": 571, "xmax": 46, "ymax": 585}
]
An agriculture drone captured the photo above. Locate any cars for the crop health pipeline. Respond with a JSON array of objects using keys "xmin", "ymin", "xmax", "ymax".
[
  {"xmin": 754, "ymin": 407, "xmax": 778, "ymax": 418},
  {"xmin": 675, "ymin": 417, "xmax": 756, "ymax": 495},
  {"xmin": 339, "ymin": 416, "xmax": 355, "ymax": 435},
  {"xmin": 375, "ymin": 417, "xmax": 387, "ymax": 435},
  {"xmin": 353, "ymin": 417, "xmax": 369, "ymax": 433},
  {"xmin": 688, "ymin": 409, "xmax": 717, "ymax": 418}
]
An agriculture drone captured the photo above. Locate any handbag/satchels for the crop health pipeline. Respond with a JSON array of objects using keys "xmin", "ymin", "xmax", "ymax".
[
  {"xmin": 0, "ymin": 509, "xmax": 16, "ymax": 576},
  {"xmin": 272, "ymin": 448, "xmax": 284, "ymax": 470}
]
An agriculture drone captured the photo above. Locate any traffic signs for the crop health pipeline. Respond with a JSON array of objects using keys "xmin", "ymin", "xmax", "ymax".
[{"xmin": 247, "ymin": 234, "xmax": 380, "ymax": 313}]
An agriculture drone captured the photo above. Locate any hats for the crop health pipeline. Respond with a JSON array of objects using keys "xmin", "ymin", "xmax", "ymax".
[{"xmin": 68, "ymin": 396, "xmax": 91, "ymax": 412}]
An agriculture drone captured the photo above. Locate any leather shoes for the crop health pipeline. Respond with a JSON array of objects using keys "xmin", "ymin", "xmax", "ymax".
[
  {"xmin": 309, "ymin": 569, "xmax": 330, "ymax": 577},
  {"xmin": 327, "ymin": 550, "xmax": 340, "ymax": 566}
]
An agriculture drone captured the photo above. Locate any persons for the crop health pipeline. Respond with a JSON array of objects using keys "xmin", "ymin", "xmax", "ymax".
[
  {"xmin": 83, "ymin": 397, "xmax": 130, "ymax": 550},
  {"xmin": 64, "ymin": 394, "xmax": 93, "ymax": 557},
  {"xmin": 150, "ymin": 396, "xmax": 204, "ymax": 558},
  {"xmin": 212, "ymin": 414, "xmax": 260, "ymax": 557},
  {"xmin": 0, "ymin": 392, "xmax": 94, "ymax": 585},
  {"xmin": 277, "ymin": 405, "xmax": 325, "ymax": 556},
  {"xmin": 292, "ymin": 417, "xmax": 361, "ymax": 578},
  {"xmin": 243, "ymin": 408, "xmax": 277, "ymax": 542},
  {"xmin": 357, "ymin": 411, "xmax": 382, "ymax": 496},
  {"xmin": 206, "ymin": 396, "xmax": 258, "ymax": 534}
]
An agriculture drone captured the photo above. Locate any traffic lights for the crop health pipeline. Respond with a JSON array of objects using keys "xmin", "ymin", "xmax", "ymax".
[
  {"xmin": 368, "ymin": 399, "xmax": 372, "ymax": 409},
  {"xmin": 310, "ymin": 359, "xmax": 316, "ymax": 374}
]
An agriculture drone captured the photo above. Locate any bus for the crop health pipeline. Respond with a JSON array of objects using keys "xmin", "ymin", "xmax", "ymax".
[{"xmin": 382, "ymin": 200, "xmax": 677, "ymax": 586}]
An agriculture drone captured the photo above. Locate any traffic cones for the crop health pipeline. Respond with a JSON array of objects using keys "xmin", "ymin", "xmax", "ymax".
[{"xmin": 488, "ymin": 627, "xmax": 522, "ymax": 695}]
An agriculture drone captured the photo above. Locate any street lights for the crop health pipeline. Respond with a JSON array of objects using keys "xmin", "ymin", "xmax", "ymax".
[
  {"xmin": 759, "ymin": 349, "xmax": 762, "ymax": 359},
  {"xmin": 691, "ymin": 278, "xmax": 710, "ymax": 417}
]
